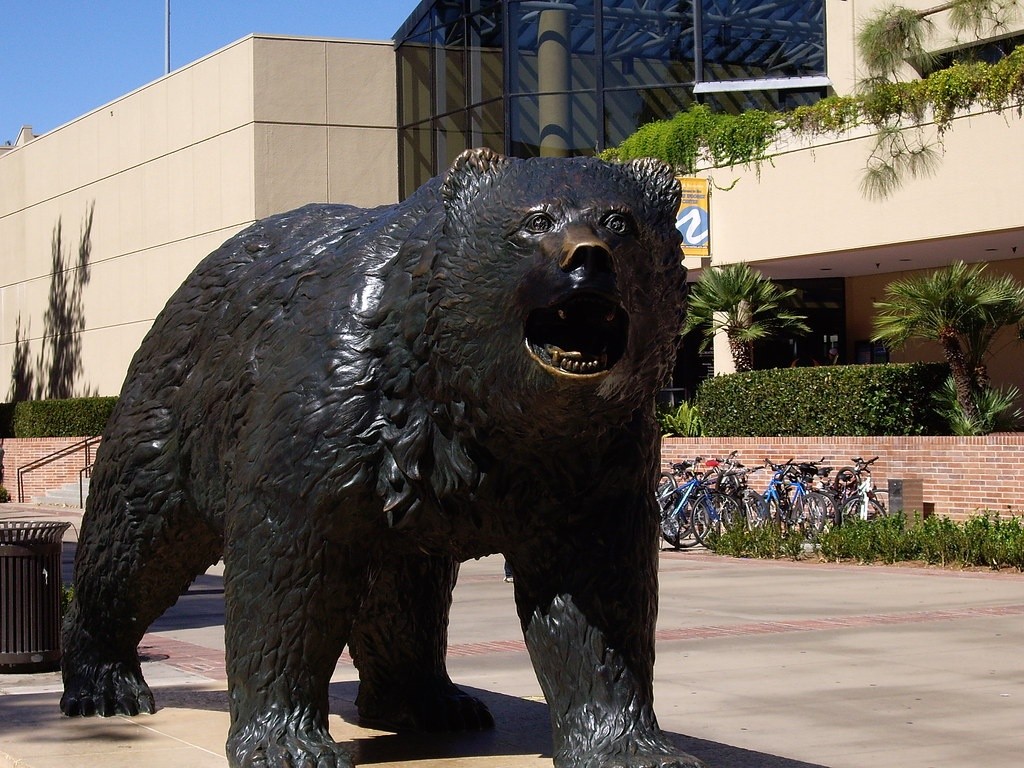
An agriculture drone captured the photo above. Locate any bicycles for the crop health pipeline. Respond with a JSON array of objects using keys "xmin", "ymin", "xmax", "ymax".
[{"xmin": 656, "ymin": 449, "xmax": 894, "ymax": 550}]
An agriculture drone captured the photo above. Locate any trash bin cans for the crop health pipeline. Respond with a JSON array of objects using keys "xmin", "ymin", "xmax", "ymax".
[{"xmin": 0, "ymin": 520, "xmax": 73, "ymax": 665}]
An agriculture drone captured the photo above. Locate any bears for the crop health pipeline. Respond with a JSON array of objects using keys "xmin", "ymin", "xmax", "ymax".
[{"xmin": 61, "ymin": 146, "xmax": 717, "ymax": 768}]
